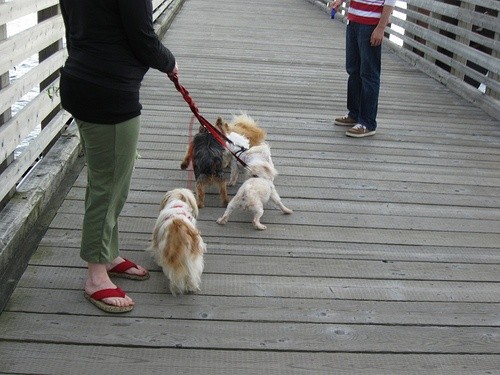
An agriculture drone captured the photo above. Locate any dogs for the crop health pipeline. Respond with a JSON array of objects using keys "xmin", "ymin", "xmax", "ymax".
[
  {"xmin": 181, "ymin": 116, "xmax": 229, "ymax": 208},
  {"xmin": 146, "ymin": 188, "xmax": 208, "ymax": 296},
  {"xmin": 216, "ymin": 110, "xmax": 293, "ymax": 230}
]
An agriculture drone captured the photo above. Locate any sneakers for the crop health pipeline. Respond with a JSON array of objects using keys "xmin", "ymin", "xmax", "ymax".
[
  {"xmin": 333, "ymin": 115, "xmax": 358, "ymax": 126},
  {"xmin": 344, "ymin": 122, "xmax": 375, "ymax": 138}
]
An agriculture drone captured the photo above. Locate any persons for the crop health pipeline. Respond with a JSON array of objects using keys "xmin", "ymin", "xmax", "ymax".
[
  {"xmin": 60, "ymin": 0, "xmax": 179, "ymax": 313},
  {"xmin": 330, "ymin": 0, "xmax": 396, "ymax": 138}
]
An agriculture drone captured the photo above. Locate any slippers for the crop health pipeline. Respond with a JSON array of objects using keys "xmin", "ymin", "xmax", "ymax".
[
  {"xmin": 107, "ymin": 256, "xmax": 150, "ymax": 280},
  {"xmin": 83, "ymin": 285, "xmax": 136, "ymax": 314}
]
